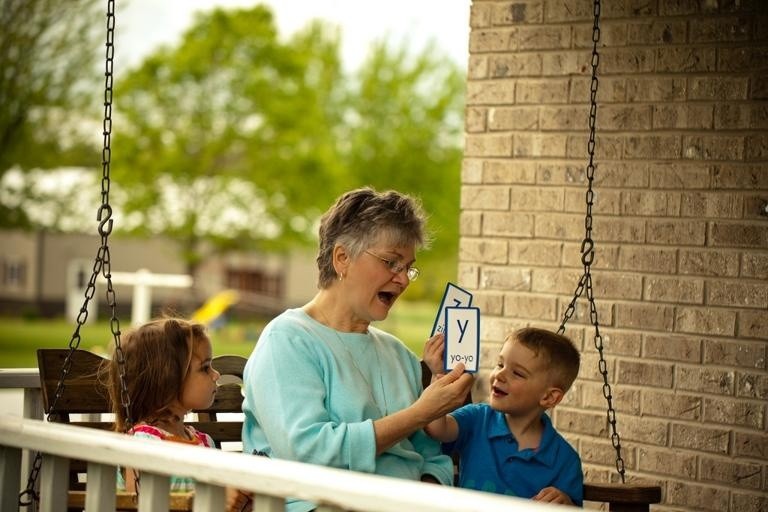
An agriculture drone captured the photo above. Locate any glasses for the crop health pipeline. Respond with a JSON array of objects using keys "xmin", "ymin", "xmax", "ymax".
[{"xmin": 356, "ymin": 246, "xmax": 419, "ymax": 282}]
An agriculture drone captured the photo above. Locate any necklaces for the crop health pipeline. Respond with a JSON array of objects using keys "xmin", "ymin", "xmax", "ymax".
[{"xmin": 312, "ymin": 299, "xmax": 389, "ymax": 420}]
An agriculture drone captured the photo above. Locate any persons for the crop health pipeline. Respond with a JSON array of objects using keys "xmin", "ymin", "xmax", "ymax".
[
  {"xmin": 423, "ymin": 327, "xmax": 583, "ymax": 506},
  {"xmin": 240, "ymin": 187, "xmax": 477, "ymax": 512},
  {"xmin": 106, "ymin": 319, "xmax": 254, "ymax": 512}
]
